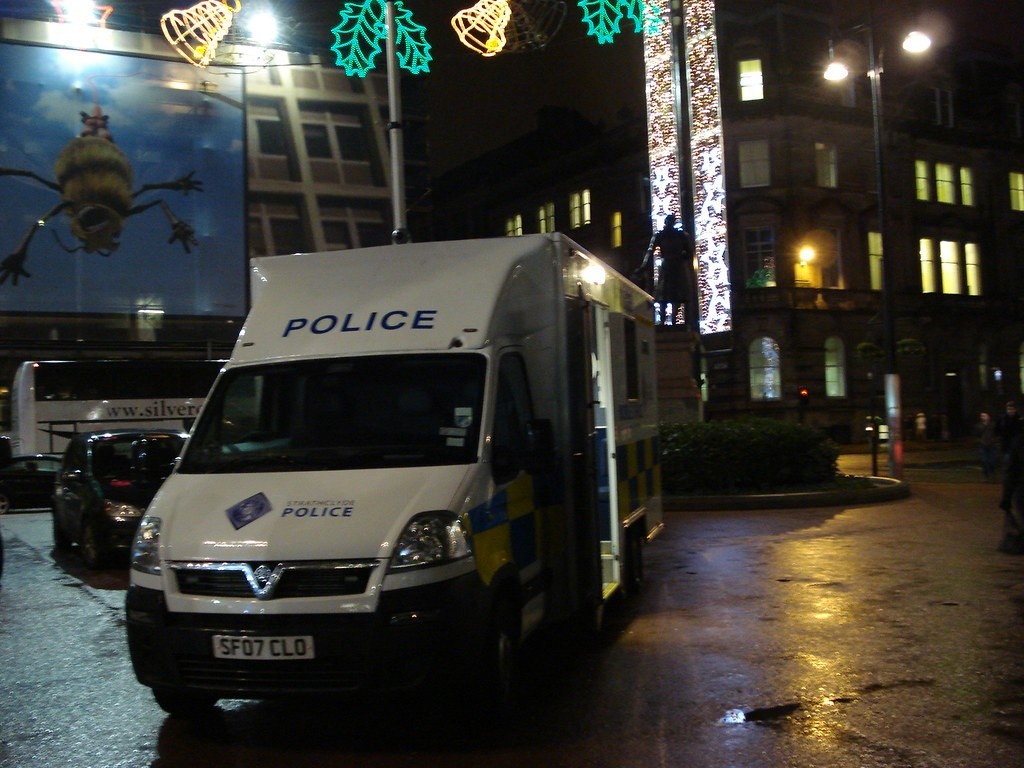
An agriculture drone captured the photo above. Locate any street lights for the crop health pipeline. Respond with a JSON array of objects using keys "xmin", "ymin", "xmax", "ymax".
[
  {"xmin": 788, "ymin": 246, "xmax": 818, "ymax": 420},
  {"xmin": 822, "ymin": 14, "xmax": 933, "ymax": 481}
]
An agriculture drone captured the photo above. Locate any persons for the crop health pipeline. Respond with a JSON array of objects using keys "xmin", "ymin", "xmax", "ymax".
[
  {"xmin": 999, "ymin": 401, "xmax": 1024, "ymax": 513},
  {"xmin": 639, "ymin": 215, "xmax": 694, "ymax": 326},
  {"xmin": 975, "ymin": 411, "xmax": 997, "ymax": 482}
]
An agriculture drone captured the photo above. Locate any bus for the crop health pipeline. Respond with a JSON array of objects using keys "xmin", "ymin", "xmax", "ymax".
[{"xmin": 10, "ymin": 359, "xmax": 225, "ymax": 503}]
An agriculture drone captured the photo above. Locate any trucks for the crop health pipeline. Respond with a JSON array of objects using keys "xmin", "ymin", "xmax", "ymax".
[{"xmin": 125, "ymin": 233, "xmax": 662, "ymax": 718}]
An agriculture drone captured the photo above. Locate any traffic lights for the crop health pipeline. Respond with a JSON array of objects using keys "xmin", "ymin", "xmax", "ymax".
[{"xmin": 799, "ymin": 387, "xmax": 808, "ymax": 396}]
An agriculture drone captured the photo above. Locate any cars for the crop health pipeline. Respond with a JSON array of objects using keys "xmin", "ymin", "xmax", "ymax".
[{"xmin": 50, "ymin": 428, "xmax": 185, "ymax": 573}]
